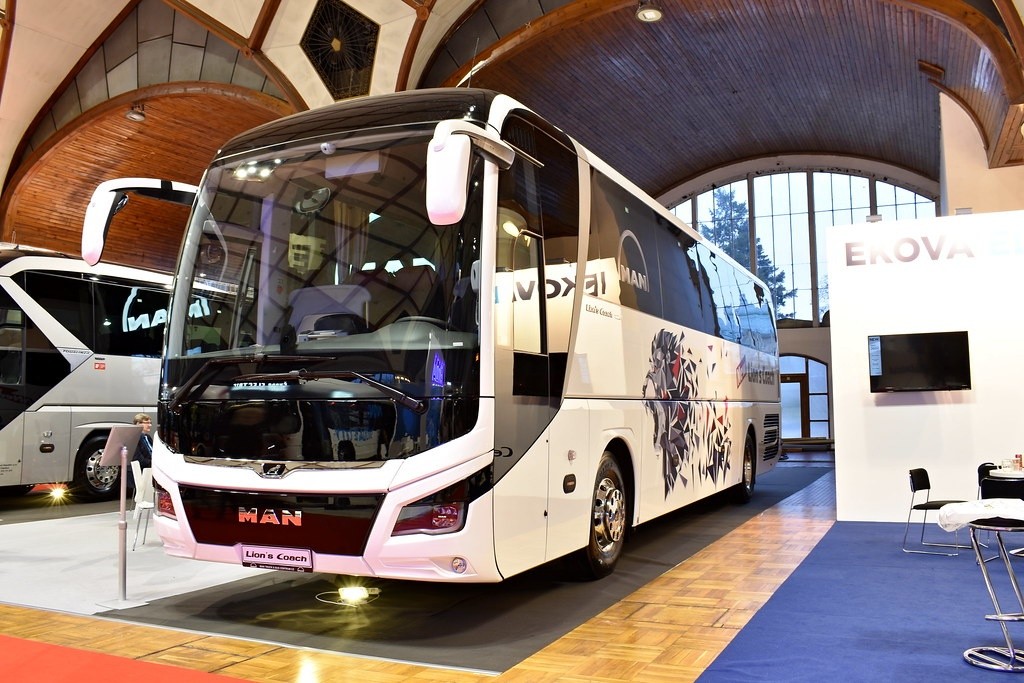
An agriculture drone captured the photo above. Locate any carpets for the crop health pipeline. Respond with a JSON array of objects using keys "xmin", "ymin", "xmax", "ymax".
[{"xmin": 692, "ymin": 521, "xmax": 1024, "ymax": 682}]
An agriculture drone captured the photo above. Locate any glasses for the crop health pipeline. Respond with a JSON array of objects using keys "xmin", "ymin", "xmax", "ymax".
[{"xmin": 139, "ymin": 421, "xmax": 152, "ymax": 425}]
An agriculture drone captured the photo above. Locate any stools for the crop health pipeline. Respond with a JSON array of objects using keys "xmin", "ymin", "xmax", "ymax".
[{"xmin": 962, "ymin": 517, "xmax": 1024, "ymax": 671}]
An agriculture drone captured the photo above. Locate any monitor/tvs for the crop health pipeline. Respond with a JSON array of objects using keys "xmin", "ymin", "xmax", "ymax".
[{"xmin": 867, "ymin": 331, "xmax": 973, "ymax": 393}]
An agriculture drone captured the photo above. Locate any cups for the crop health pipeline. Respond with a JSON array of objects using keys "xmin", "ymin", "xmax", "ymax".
[
  {"xmin": 997, "ymin": 460, "xmax": 1013, "ymax": 473},
  {"xmin": 1012, "ymin": 458, "xmax": 1020, "ymax": 472}
]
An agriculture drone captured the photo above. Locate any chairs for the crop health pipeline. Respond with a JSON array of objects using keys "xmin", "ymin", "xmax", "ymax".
[
  {"xmin": 902, "ymin": 468, "xmax": 975, "ymax": 556},
  {"xmin": 977, "ymin": 462, "xmax": 996, "ymax": 500}
]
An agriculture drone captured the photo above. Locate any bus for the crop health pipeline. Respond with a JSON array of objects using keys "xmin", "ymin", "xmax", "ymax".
[
  {"xmin": 78, "ymin": 86, "xmax": 786, "ymax": 588},
  {"xmin": 0, "ymin": 240, "xmax": 255, "ymax": 500}
]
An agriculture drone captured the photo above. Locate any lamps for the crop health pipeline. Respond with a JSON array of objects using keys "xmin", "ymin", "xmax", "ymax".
[{"xmin": 635, "ymin": 0, "xmax": 665, "ymax": 24}]
